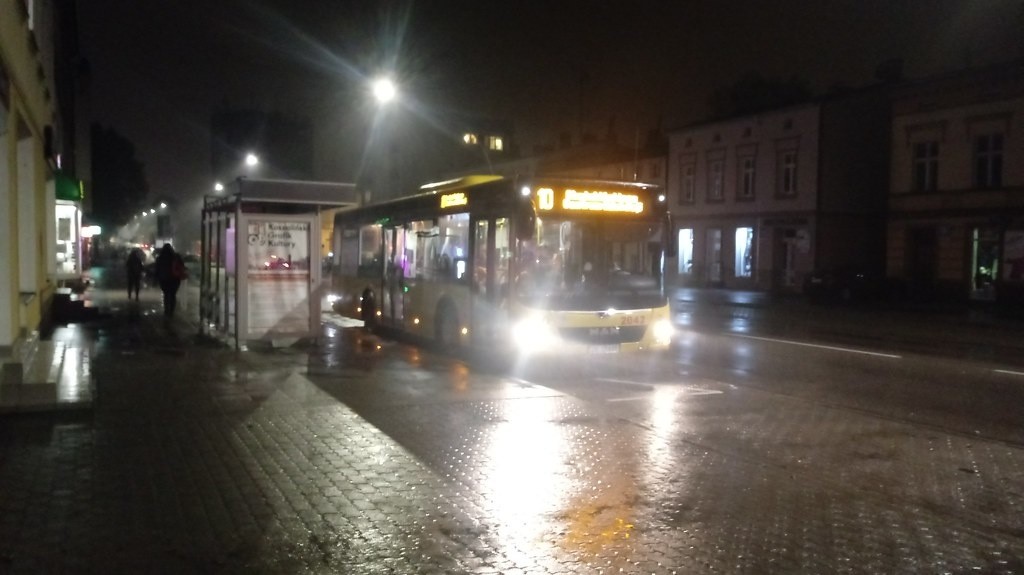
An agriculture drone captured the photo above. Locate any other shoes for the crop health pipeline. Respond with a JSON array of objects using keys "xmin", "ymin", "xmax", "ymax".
[
  {"xmin": 165, "ymin": 309, "xmax": 175, "ymax": 314},
  {"xmin": 135, "ymin": 297, "xmax": 139, "ymax": 301},
  {"xmin": 128, "ymin": 294, "xmax": 131, "ymax": 299}
]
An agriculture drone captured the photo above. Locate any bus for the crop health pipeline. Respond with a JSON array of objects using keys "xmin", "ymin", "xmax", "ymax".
[{"xmin": 328, "ymin": 174, "xmax": 676, "ymax": 365}]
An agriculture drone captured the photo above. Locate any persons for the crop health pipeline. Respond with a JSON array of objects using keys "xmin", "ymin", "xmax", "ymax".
[
  {"xmin": 154, "ymin": 243, "xmax": 190, "ymax": 319},
  {"xmin": 124, "ymin": 245, "xmax": 147, "ymax": 300}
]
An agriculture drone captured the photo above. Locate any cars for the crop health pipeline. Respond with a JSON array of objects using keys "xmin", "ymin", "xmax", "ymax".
[
  {"xmin": 110, "ymin": 241, "xmax": 158, "ymax": 263},
  {"xmin": 142, "ymin": 252, "xmax": 202, "ymax": 278},
  {"xmin": 802, "ymin": 254, "xmax": 911, "ymax": 306},
  {"xmin": 321, "ymin": 253, "xmax": 333, "ymax": 272}
]
